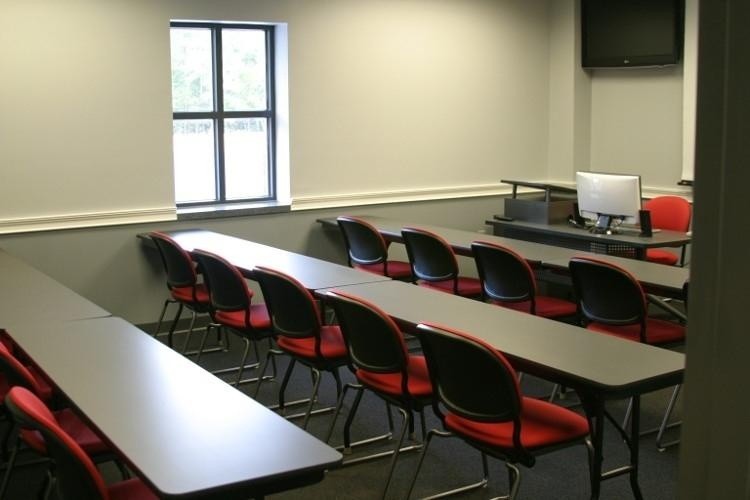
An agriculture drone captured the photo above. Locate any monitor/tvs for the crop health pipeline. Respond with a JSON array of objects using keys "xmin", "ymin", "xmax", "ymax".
[
  {"xmin": 575, "ymin": 171, "xmax": 641, "ymax": 234},
  {"xmin": 580, "ymin": 1, "xmax": 684, "ymax": 72}
]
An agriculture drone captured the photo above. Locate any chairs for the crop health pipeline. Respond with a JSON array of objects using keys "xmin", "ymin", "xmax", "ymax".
[{"xmin": 0, "ymin": 195, "xmax": 695, "ymax": 499}]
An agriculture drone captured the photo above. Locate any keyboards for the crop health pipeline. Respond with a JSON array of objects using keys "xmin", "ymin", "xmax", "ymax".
[{"xmin": 586, "ymin": 221, "xmax": 662, "ymax": 234}]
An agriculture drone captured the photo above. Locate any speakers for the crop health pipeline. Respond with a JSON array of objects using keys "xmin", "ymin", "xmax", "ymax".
[
  {"xmin": 573, "ymin": 202, "xmax": 585, "ymax": 228},
  {"xmin": 639, "ymin": 210, "xmax": 653, "ymax": 237}
]
